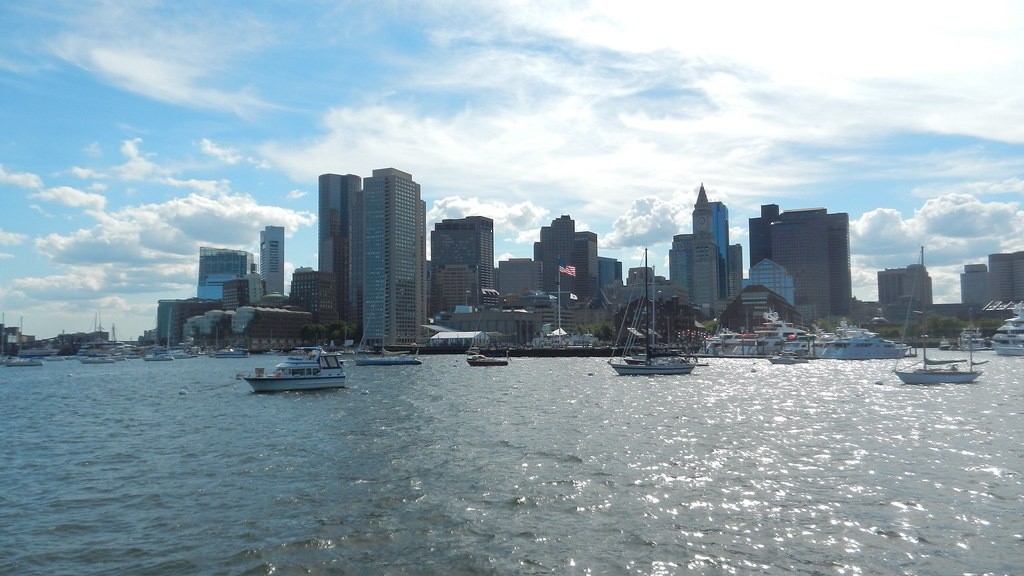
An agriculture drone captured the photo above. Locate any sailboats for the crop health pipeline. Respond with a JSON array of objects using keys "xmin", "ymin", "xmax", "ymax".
[
  {"xmin": 465, "ymin": 311, "xmax": 484, "ymax": 356},
  {"xmin": 891, "ymin": 245, "xmax": 984, "ymax": 385},
  {"xmin": 0, "ymin": 310, "xmax": 250, "ymax": 368},
  {"xmin": 608, "ymin": 248, "xmax": 698, "ymax": 376},
  {"xmin": 354, "ymin": 275, "xmax": 422, "ymax": 366}
]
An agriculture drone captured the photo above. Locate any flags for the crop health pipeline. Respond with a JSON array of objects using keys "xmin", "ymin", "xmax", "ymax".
[{"xmin": 559, "ymin": 259, "xmax": 576, "ymax": 276}]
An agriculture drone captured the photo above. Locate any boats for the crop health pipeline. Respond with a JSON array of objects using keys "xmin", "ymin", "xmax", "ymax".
[
  {"xmin": 957, "ymin": 324, "xmax": 989, "ymax": 350},
  {"xmin": 769, "ymin": 349, "xmax": 809, "ymax": 365},
  {"xmin": 275, "ymin": 345, "xmax": 345, "ymax": 370},
  {"xmin": 244, "ymin": 352, "xmax": 347, "ymax": 392},
  {"xmin": 382, "ymin": 347, "xmax": 410, "ymax": 357},
  {"xmin": 466, "ymin": 353, "xmax": 509, "ymax": 367},
  {"xmin": 939, "ymin": 339, "xmax": 950, "ymax": 350},
  {"xmin": 696, "ymin": 309, "xmax": 912, "ymax": 362},
  {"xmin": 988, "ymin": 301, "xmax": 1024, "ymax": 356}
]
